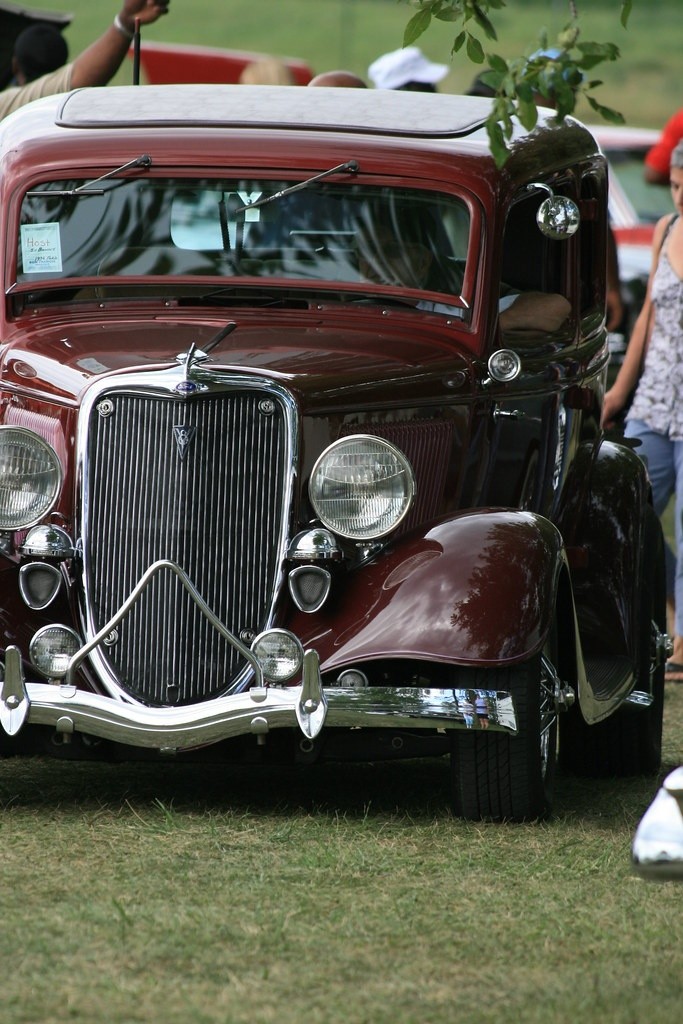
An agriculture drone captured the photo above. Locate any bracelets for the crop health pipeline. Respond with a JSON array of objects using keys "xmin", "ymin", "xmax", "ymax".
[{"xmin": 114, "ymin": 14, "xmax": 135, "ymax": 39}]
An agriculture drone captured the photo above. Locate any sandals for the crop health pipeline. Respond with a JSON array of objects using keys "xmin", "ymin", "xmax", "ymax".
[{"xmin": 665, "ymin": 661, "xmax": 683, "ymax": 684}]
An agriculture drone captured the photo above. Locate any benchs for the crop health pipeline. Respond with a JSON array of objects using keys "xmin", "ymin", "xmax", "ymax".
[{"xmin": 98, "ymin": 246, "xmax": 434, "ymax": 289}]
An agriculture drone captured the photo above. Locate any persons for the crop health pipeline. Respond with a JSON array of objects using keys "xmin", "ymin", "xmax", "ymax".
[{"xmin": 0, "ymin": 0, "xmax": 682, "ymax": 677}]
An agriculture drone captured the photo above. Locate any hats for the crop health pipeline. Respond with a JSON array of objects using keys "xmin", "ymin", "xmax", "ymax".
[
  {"xmin": 368, "ymin": 46, "xmax": 449, "ymax": 89},
  {"xmin": 466, "ymin": 69, "xmax": 505, "ymax": 99},
  {"xmin": 523, "ymin": 48, "xmax": 586, "ymax": 92}
]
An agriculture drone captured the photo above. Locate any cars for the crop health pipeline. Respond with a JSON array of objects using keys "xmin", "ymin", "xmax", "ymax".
[{"xmin": 0, "ymin": 41, "xmax": 675, "ymax": 828}]
